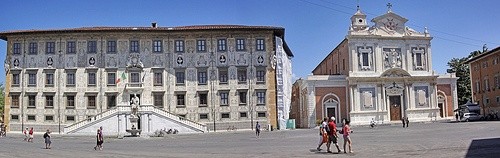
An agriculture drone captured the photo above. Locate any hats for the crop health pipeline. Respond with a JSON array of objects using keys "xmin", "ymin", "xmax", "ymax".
[{"xmin": 331, "ymin": 117, "xmax": 335, "ymax": 121}]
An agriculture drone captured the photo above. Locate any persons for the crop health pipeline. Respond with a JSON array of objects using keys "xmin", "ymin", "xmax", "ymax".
[
  {"xmin": 343, "ymin": 119, "xmax": 353, "ymax": 154},
  {"xmin": 256, "ymin": 121, "xmax": 260, "ymax": 136},
  {"xmin": 456, "ymin": 113, "xmax": 458, "ymax": 122},
  {"xmin": 130, "ymin": 93, "xmax": 139, "ymax": 114},
  {"xmin": 370, "ymin": 118, "xmax": 376, "ymax": 128},
  {"xmin": 402, "ymin": 117, "xmax": 405, "ymax": 127},
  {"xmin": 94, "ymin": 129, "xmax": 102, "ymax": 150},
  {"xmin": 44, "ymin": 129, "xmax": 51, "ymax": 149},
  {"xmin": 318, "ymin": 121, "xmax": 324, "ymax": 146},
  {"xmin": 98, "ymin": 126, "xmax": 103, "ymax": 149},
  {"xmin": 406, "ymin": 117, "xmax": 409, "ymax": 127},
  {"xmin": 317, "ymin": 117, "xmax": 329, "ymax": 151},
  {"xmin": 327, "ymin": 117, "xmax": 342, "ymax": 153},
  {"xmin": 28, "ymin": 127, "xmax": 33, "ymax": 142},
  {"xmin": 24, "ymin": 127, "xmax": 29, "ymax": 142},
  {"xmin": 0, "ymin": 123, "xmax": 7, "ymax": 138}
]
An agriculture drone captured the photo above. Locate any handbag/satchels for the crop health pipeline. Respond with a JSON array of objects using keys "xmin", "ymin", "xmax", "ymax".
[{"xmin": 339, "ymin": 128, "xmax": 344, "ymax": 134}]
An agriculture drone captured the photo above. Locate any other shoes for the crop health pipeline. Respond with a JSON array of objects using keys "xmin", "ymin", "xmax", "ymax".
[
  {"xmin": 338, "ymin": 150, "xmax": 341, "ymax": 152},
  {"xmin": 350, "ymin": 151, "xmax": 353, "ymax": 153},
  {"xmin": 328, "ymin": 150, "xmax": 332, "ymax": 153},
  {"xmin": 317, "ymin": 147, "xmax": 321, "ymax": 151},
  {"xmin": 344, "ymin": 151, "xmax": 347, "ymax": 153}
]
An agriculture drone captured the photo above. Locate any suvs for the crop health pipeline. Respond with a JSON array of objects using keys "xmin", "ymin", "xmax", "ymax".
[{"xmin": 463, "ymin": 112, "xmax": 484, "ymax": 122}]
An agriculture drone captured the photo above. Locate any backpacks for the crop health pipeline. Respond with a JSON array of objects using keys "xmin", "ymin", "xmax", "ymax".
[{"xmin": 325, "ymin": 123, "xmax": 330, "ymax": 132}]
